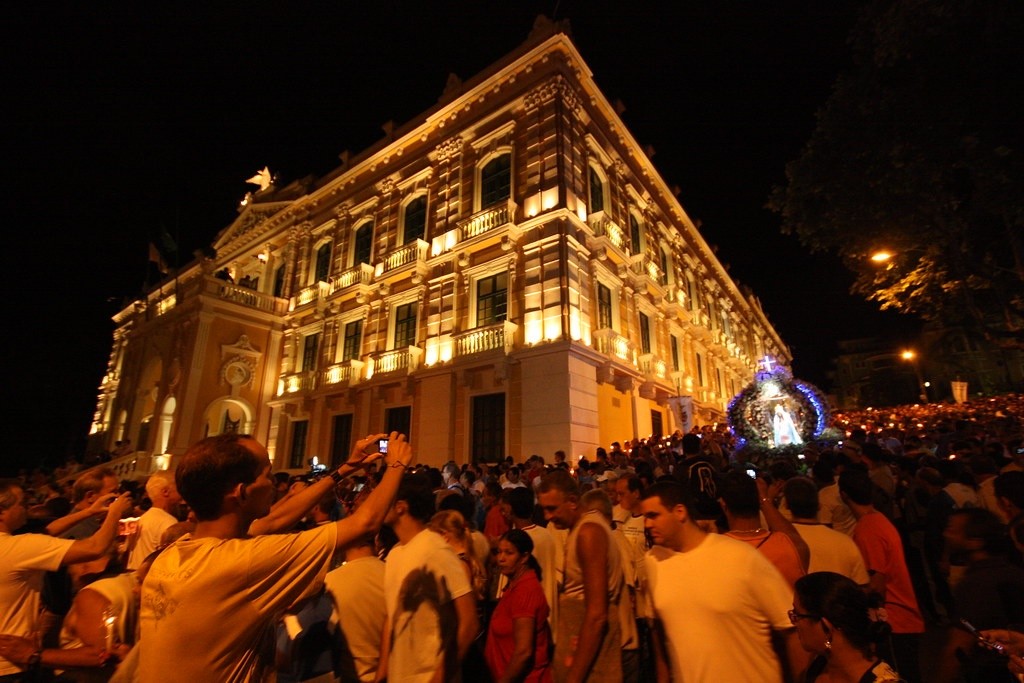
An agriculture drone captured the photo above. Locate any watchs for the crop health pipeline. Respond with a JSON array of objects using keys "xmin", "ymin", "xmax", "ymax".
[{"xmin": 27, "ymin": 648, "xmax": 46, "ymax": 671}]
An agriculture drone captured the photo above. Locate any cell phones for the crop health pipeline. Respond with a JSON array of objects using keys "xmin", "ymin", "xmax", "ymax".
[
  {"xmin": 838, "ymin": 440, "xmax": 843, "ymax": 449},
  {"xmin": 959, "ymin": 617, "xmax": 1004, "ymax": 651},
  {"xmin": 745, "ymin": 466, "xmax": 757, "ymax": 481}
]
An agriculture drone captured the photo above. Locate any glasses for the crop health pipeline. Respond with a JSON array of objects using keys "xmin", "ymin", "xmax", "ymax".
[{"xmin": 787, "ymin": 610, "xmax": 818, "ymax": 625}]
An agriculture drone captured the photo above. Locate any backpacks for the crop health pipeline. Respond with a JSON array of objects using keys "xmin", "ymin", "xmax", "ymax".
[{"xmin": 450, "ymin": 485, "xmax": 475, "ymax": 519}]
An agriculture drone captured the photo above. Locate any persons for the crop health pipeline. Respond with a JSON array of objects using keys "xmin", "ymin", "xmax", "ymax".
[
  {"xmin": 238, "ymin": 275, "xmax": 259, "ymax": 305},
  {"xmin": 0, "ymin": 394, "xmax": 1024, "ymax": 683},
  {"xmin": 214, "ymin": 266, "xmax": 235, "ymax": 292}
]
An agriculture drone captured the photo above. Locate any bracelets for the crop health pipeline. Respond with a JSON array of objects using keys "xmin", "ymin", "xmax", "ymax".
[
  {"xmin": 329, "ymin": 469, "xmax": 343, "ymax": 483},
  {"xmin": 760, "ymin": 496, "xmax": 771, "ymax": 505}
]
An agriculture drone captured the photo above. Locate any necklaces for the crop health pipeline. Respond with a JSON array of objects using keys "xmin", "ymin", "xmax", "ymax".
[
  {"xmin": 728, "ymin": 528, "xmax": 767, "ymax": 533},
  {"xmin": 857, "ymin": 509, "xmax": 880, "ymax": 521},
  {"xmin": 511, "ymin": 565, "xmax": 528, "ymax": 579}
]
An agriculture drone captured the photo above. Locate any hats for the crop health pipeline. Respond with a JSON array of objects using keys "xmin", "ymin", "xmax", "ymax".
[{"xmin": 597, "ymin": 471, "xmax": 618, "ymax": 482}]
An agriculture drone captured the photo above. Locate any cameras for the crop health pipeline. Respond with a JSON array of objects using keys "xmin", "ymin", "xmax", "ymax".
[
  {"xmin": 378, "ymin": 438, "xmax": 389, "ymax": 454},
  {"xmin": 1016, "ymin": 448, "xmax": 1024, "ymax": 454},
  {"xmin": 796, "ymin": 454, "xmax": 806, "ymax": 460}
]
